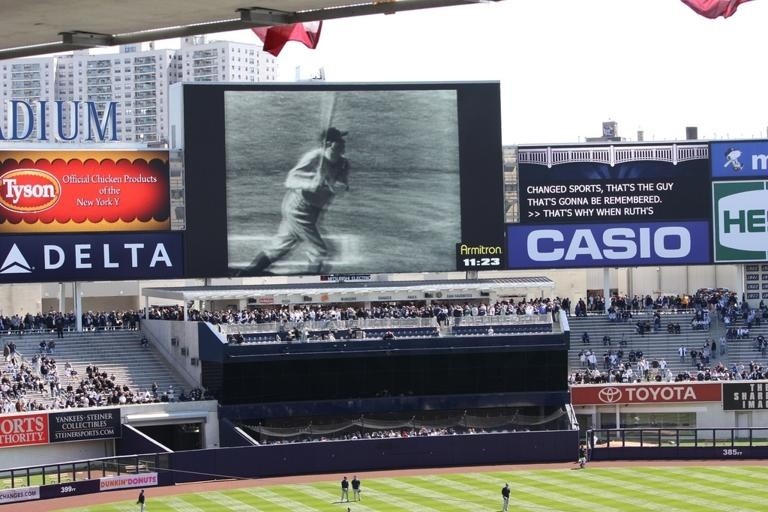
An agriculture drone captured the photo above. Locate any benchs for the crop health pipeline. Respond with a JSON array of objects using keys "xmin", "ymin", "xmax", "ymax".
[
  {"xmin": 0, "ymin": 327, "xmax": 196, "ymax": 414},
  {"xmin": 566, "ymin": 303, "xmax": 768, "ymax": 387}
]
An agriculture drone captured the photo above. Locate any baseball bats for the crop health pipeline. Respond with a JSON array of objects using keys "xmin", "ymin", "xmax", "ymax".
[{"xmin": 316, "ymin": 91, "xmax": 337, "ymax": 179}]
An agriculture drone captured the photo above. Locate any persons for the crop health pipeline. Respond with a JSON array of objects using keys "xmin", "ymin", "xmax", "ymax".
[
  {"xmin": 137, "ymin": 489, "xmax": 146, "ymax": 512},
  {"xmin": 241, "ymin": 128, "xmax": 352, "ymax": 276},
  {"xmin": 351, "ymin": 475, "xmax": 361, "ymax": 502},
  {"xmin": 502, "ymin": 483, "xmax": 510, "ymax": 511},
  {"xmin": 340, "ymin": 476, "xmax": 349, "ymax": 502},
  {"xmin": 578, "ymin": 445, "xmax": 587, "ymax": 469}
]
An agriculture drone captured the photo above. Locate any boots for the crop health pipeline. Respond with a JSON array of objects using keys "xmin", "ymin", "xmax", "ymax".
[{"xmin": 238, "ymin": 250, "xmax": 271, "ymax": 278}]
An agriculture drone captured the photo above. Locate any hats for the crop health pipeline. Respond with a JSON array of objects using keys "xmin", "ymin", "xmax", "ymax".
[{"xmin": 320, "ymin": 127, "xmax": 349, "ymax": 142}]
